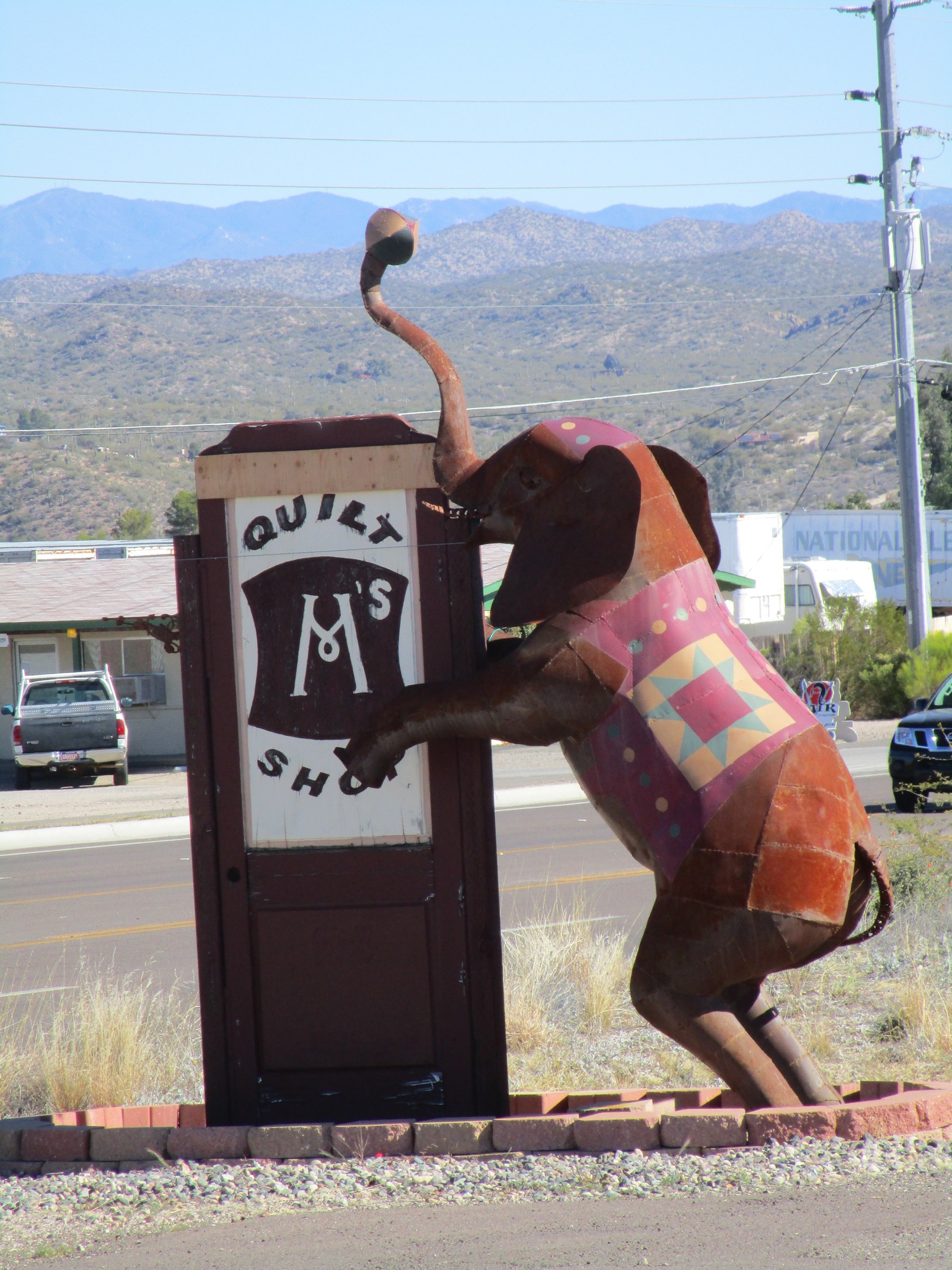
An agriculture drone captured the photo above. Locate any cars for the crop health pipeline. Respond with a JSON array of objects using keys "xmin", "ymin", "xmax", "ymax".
[{"xmin": 888, "ymin": 675, "xmax": 952, "ymax": 810}]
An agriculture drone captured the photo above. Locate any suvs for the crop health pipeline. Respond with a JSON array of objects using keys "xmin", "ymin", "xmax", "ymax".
[{"xmin": 1, "ymin": 663, "xmax": 128, "ymax": 787}]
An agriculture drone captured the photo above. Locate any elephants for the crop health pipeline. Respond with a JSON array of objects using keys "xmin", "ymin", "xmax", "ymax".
[{"xmin": 342, "ymin": 249, "xmax": 893, "ymax": 1112}]
